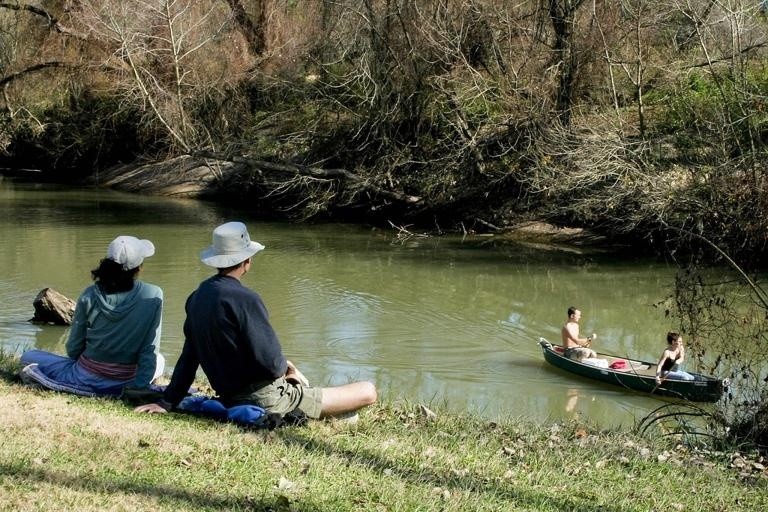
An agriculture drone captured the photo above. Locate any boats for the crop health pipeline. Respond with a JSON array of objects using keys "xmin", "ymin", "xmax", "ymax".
[{"xmin": 538, "ymin": 338, "xmax": 724, "ymax": 403}]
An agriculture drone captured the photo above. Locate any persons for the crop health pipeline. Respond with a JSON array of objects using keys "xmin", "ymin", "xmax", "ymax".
[
  {"xmin": 19, "ymin": 235, "xmax": 165, "ymax": 398},
  {"xmin": 562, "ymin": 307, "xmax": 597, "ymax": 360},
  {"xmin": 655, "ymin": 331, "xmax": 695, "ymax": 385},
  {"xmin": 132, "ymin": 221, "xmax": 377, "ymax": 429}
]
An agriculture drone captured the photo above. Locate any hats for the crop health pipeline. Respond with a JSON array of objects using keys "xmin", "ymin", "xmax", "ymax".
[
  {"xmin": 199, "ymin": 220, "xmax": 266, "ymax": 268},
  {"xmin": 106, "ymin": 235, "xmax": 156, "ymax": 272}
]
